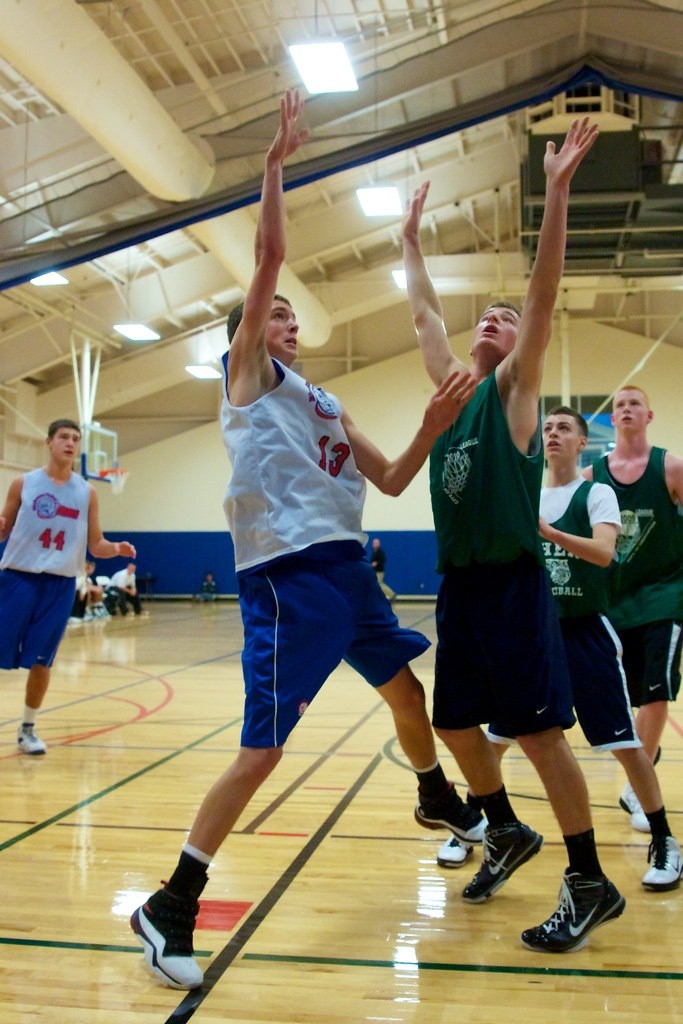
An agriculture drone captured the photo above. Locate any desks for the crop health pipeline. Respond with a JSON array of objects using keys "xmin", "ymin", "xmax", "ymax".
[{"xmin": 136, "ymin": 575, "xmax": 156, "ymax": 602}]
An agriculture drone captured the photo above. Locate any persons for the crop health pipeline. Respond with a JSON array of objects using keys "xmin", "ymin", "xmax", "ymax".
[
  {"xmin": 435, "ymin": 408, "xmax": 683, "ymax": 891},
  {"xmin": 581, "ymin": 385, "xmax": 683, "ymax": 831},
  {"xmin": 0, "ymin": 420, "xmax": 137, "ymax": 755},
  {"xmin": 106, "ymin": 561, "xmax": 149, "ymax": 616},
  {"xmin": 199, "ymin": 572, "xmax": 216, "ymax": 602},
  {"xmin": 128, "ymin": 87, "xmax": 490, "ymax": 992},
  {"xmin": 67, "ymin": 559, "xmax": 104, "ymax": 625},
  {"xmin": 404, "ymin": 115, "xmax": 627, "ymax": 955},
  {"xmin": 369, "ymin": 538, "xmax": 395, "ymax": 602}
]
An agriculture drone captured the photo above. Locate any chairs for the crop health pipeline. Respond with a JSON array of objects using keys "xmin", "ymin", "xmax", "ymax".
[{"xmin": 86, "ymin": 576, "xmax": 111, "ymax": 616}]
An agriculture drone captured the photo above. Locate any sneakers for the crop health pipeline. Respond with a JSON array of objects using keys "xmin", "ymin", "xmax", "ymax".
[
  {"xmin": 437, "ymin": 828, "xmax": 473, "ymax": 867},
  {"xmin": 17, "ymin": 731, "xmax": 46, "ymax": 754},
  {"xmin": 415, "ymin": 781, "xmax": 489, "ymax": 845},
  {"xmin": 520, "ymin": 864, "xmax": 626, "ymax": 955},
  {"xmin": 619, "ymin": 781, "xmax": 653, "ymax": 833},
  {"xmin": 642, "ymin": 834, "xmax": 683, "ymax": 890},
  {"xmin": 130, "ymin": 880, "xmax": 204, "ymax": 989},
  {"xmin": 462, "ymin": 820, "xmax": 543, "ymax": 903}
]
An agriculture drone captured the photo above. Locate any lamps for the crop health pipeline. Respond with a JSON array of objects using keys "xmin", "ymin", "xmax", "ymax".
[
  {"xmin": 286, "ymin": 0, "xmax": 358, "ymax": 93},
  {"xmin": 355, "ymin": 160, "xmax": 403, "ymax": 217},
  {"xmin": 111, "ymin": 248, "xmax": 162, "ymax": 341},
  {"xmin": 182, "ymin": 295, "xmax": 224, "ymax": 379}
]
